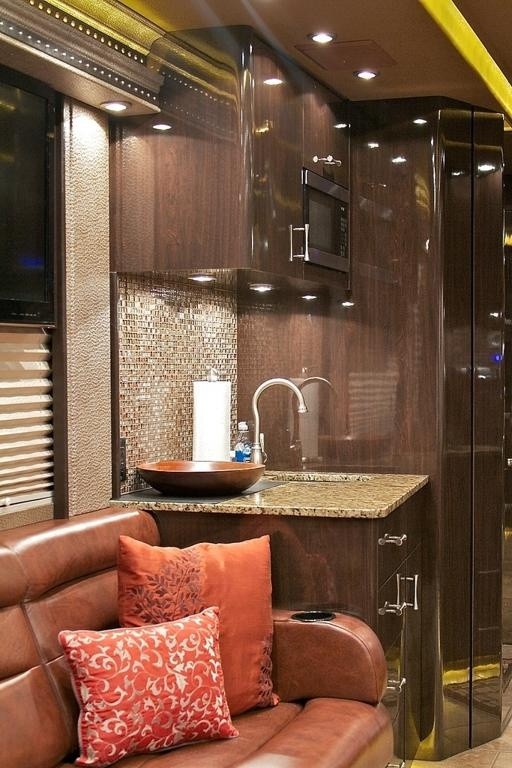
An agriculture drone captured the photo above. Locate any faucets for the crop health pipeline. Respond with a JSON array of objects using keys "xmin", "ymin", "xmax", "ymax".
[
  {"xmin": 292, "ymin": 376, "xmax": 339, "ymax": 446},
  {"xmin": 252, "ymin": 379, "xmax": 308, "ymax": 447}
]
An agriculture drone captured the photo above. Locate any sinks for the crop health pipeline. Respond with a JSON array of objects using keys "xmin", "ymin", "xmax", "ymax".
[{"xmin": 267, "ymin": 473, "xmax": 377, "ymax": 483}]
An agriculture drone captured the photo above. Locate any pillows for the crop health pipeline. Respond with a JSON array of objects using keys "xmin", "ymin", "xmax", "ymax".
[
  {"xmin": 57, "ymin": 606, "xmax": 240, "ymax": 768},
  {"xmin": 118, "ymin": 534, "xmax": 281, "ymax": 717}
]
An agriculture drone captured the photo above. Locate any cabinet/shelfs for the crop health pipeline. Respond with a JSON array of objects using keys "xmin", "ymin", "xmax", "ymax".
[
  {"xmin": 148, "ymin": 491, "xmax": 440, "ymax": 762},
  {"xmin": 110, "ymin": 24, "xmax": 350, "ymax": 291}
]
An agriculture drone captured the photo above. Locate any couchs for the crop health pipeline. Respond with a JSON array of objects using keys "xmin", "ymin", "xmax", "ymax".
[{"xmin": 1, "ymin": 506, "xmax": 394, "ymax": 768}]
